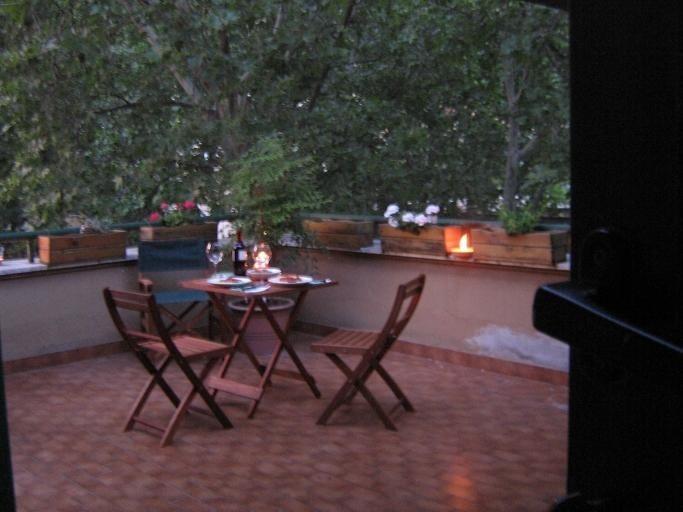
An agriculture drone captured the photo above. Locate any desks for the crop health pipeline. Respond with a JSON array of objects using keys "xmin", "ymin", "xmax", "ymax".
[{"xmin": 179, "ymin": 273, "xmax": 339, "ymax": 418}]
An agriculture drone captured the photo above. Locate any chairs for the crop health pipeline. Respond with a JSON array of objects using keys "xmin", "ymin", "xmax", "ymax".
[
  {"xmin": 102, "ymin": 286, "xmax": 235, "ymax": 448},
  {"xmin": 138, "ymin": 241, "xmax": 213, "ymax": 339},
  {"xmin": 309, "ymin": 273, "xmax": 426, "ymax": 430}
]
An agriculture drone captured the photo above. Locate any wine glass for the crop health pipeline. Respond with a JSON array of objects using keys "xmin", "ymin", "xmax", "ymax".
[
  {"xmin": 250, "ymin": 242, "xmax": 272, "ymax": 284},
  {"xmin": 205, "ymin": 240, "xmax": 226, "ymax": 279}
]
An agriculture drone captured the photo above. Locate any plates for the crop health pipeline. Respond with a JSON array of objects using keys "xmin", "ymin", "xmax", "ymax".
[
  {"xmin": 246, "ymin": 268, "xmax": 282, "ymax": 280},
  {"xmin": 268, "ymin": 276, "xmax": 312, "ymax": 286},
  {"xmin": 206, "ymin": 276, "xmax": 252, "ymax": 287},
  {"xmin": 451, "ymin": 248, "xmax": 474, "ymax": 259}
]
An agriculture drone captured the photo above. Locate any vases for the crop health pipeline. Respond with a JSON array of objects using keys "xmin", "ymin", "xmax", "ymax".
[
  {"xmin": 378, "ymin": 224, "xmax": 481, "ymax": 257},
  {"xmin": 139, "ymin": 222, "xmax": 217, "ymax": 241}
]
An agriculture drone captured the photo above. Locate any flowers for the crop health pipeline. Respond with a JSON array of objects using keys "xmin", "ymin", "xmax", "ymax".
[
  {"xmin": 145, "ymin": 201, "xmax": 212, "ymax": 227},
  {"xmin": 383, "ymin": 203, "xmax": 441, "ymax": 236}
]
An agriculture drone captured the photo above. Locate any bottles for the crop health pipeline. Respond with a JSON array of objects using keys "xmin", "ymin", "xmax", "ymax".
[{"xmin": 232, "ymin": 230, "xmax": 249, "ymax": 276}]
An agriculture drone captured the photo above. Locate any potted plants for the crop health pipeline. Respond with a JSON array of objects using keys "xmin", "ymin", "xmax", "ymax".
[
  {"xmin": 471, "ymin": 183, "xmax": 570, "ymax": 268},
  {"xmin": 218, "ymin": 130, "xmax": 333, "ymax": 354}
]
